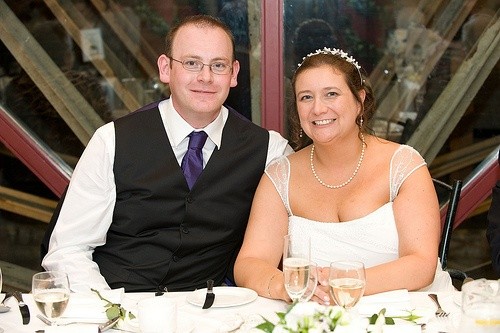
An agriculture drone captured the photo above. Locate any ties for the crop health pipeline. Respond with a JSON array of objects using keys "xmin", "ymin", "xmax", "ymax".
[{"xmin": 180, "ymin": 131, "xmax": 208, "ymax": 190}]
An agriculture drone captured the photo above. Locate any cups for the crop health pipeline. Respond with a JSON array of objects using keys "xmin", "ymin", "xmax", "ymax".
[
  {"xmin": 282, "ymin": 261, "xmax": 318, "ymax": 302},
  {"xmin": 283, "ymin": 233, "xmax": 311, "ymax": 302},
  {"xmin": 461, "ymin": 279, "xmax": 500, "ymax": 333},
  {"xmin": 137, "ymin": 298, "xmax": 178, "ymax": 333},
  {"xmin": 330, "ymin": 262, "xmax": 364, "ymax": 311}
]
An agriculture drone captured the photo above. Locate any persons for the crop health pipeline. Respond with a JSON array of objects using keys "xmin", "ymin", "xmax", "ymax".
[
  {"xmin": 39, "ymin": 16, "xmax": 295, "ymax": 293},
  {"xmin": 293, "ymin": 18, "xmax": 337, "ymax": 60},
  {"xmin": 234, "ymin": 47, "xmax": 458, "ymax": 305},
  {"xmin": 0, "ymin": 18, "xmax": 115, "ymax": 158}
]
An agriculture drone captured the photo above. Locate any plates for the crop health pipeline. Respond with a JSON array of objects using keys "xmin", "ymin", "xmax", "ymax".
[
  {"xmin": 61, "ymin": 288, "xmax": 125, "ymax": 320},
  {"xmin": 187, "ymin": 286, "xmax": 259, "ymax": 307}
]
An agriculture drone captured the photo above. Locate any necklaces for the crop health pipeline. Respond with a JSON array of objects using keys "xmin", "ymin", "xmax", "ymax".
[{"xmin": 310, "ymin": 132, "xmax": 365, "ymax": 188}]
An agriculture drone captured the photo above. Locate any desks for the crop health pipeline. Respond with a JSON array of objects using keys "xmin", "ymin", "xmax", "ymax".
[{"xmin": 0, "ymin": 293, "xmax": 500, "ymax": 333}]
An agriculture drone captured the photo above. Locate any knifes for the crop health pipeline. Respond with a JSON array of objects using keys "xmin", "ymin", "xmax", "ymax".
[
  {"xmin": 13, "ymin": 291, "xmax": 30, "ymax": 325},
  {"xmin": 202, "ymin": 280, "xmax": 215, "ymax": 309}
]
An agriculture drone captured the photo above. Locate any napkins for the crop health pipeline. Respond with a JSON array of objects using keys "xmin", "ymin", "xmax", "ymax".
[
  {"xmin": 356, "ymin": 287, "xmax": 416, "ymax": 318},
  {"xmin": 58, "ymin": 287, "xmax": 125, "ymax": 326}
]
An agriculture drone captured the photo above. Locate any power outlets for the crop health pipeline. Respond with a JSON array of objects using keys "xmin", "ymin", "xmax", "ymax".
[{"xmin": 81, "ymin": 28, "xmax": 105, "ymax": 61}]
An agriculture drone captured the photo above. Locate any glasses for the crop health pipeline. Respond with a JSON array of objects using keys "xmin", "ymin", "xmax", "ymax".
[{"xmin": 169, "ymin": 57, "xmax": 234, "ymax": 75}]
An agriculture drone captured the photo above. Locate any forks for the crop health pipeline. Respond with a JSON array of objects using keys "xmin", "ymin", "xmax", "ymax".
[
  {"xmin": 35, "ymin": 314, "xmax": 109, "ymax": 325},
  {"xmin": 429, "ymin": 293, "xmax": 450, "ymax": 317}
]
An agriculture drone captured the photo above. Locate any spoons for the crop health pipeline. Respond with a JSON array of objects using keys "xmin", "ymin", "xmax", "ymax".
[
  {"xmin": 0, "ymin": 292, "xmax": 12, "ymax": 313},
  {"xmin": 31, "ymin": 271, "xmax": 70, "ymax": 333}
]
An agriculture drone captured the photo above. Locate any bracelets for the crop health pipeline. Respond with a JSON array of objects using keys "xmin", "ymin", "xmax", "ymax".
[{"xmin": 267, "ymin": 273, "xmax": 279, "ymax": 298}]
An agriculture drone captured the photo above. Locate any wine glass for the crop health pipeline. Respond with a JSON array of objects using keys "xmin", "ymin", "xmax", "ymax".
[{"xmin": 311, "ymin": 245, "xmax": 344, "ymax": 293}]
